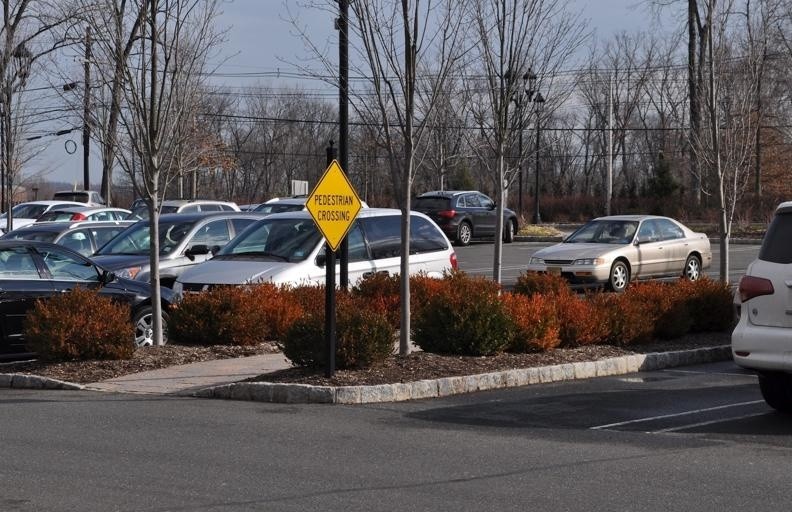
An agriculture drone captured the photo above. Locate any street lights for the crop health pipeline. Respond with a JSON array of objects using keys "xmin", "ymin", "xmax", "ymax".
[
  {"xmin": 56, "ymin": 80, "xmax": 91, "ymax": 190},
  {"xmin": 504, "ymin": 68, "xmax": 545, "ymax": 224},
  {"xmin": 0, "ymin": 42, "xmax": 33, "ymax": 232}
]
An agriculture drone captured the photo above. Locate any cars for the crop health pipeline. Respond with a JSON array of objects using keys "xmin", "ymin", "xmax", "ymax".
[
  {"xmin": 731, "ymin": 200, "xmax": 792, "ymax": 411},
  {"xmin": 527, "ymin": 214, "xmax": 713, "ymax": 292}
]
